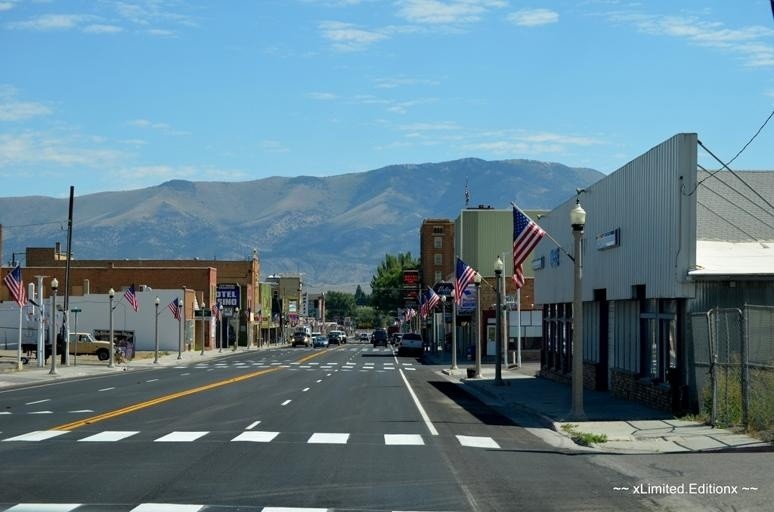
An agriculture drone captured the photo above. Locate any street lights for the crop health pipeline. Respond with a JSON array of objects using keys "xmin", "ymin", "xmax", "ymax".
[
  {"xmin": 49, "ymin": 278, "xmax": 59, "ymax": 375},
  {"xmin": 177, "ymin": 299, "xmax": 183, "ymax": 359},
  {"xmin": 494, "ymin": 255, "xmax": 504, "ymax": 382},
  {"xmin": 154, "ymin": 295, "xmax": 160, "ymax": 364},
  {"xmin": 108, "ymin": 288, "xmax": 115, "ymax": 368},
  {"xmin": 570, "ymin": 198, "xmax": 587, "ymax": 417},
  {"xmin": 441, "ymin": 269, "xmax": 481, "ymax": 376},
  {"xmin": 200, "ymin": 302, "xmax": 284, "ymax": 355}
]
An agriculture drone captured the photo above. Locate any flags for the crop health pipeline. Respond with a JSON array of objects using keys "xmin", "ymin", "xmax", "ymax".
[
  {"xmin": 211, "ymin": 300, "xmax": 221, "ymax": 318},
  {"xmin": 168, "ymin": 298, "xmax": 181, "ymax": 320},
  {"xmin": 391, "ymin": 284, "xmax": 440, "ymax": 323},
  {"xmin": 451, "ymin": 256, "xmax": 485, "ymax": 305},
  {"xmin": 510, "ymin": 203, "xmax": 547, "ymax": 290},
  {"xmin": 124, "ymin": 282, "xmax": 141, "ymax": 313},
  {"xmin": 2, "ymin": 260, "xmax": 29, "ymax": 307}
]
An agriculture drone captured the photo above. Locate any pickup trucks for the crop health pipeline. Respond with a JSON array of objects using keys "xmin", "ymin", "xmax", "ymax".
[{"xmin": 21, "ymin": 332, "xmax": 125, "ymax": 364}]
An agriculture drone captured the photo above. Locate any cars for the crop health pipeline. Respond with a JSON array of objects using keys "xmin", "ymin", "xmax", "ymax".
[
  {"xmin": 359, "ymin": 326, "xmax": 424, "ymax": 356},
  {"xmin": 290, "ymin": 324, "xmax": 347, "ymax": 348}
]
